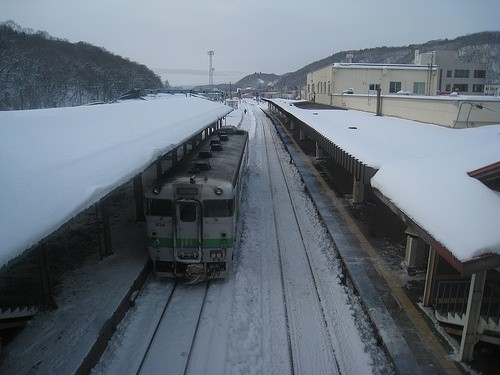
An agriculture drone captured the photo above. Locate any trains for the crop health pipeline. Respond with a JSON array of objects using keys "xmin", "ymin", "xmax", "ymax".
[{"xmin": 144, "ymin": 125, "xmax": 249, "ymax": 288}]
